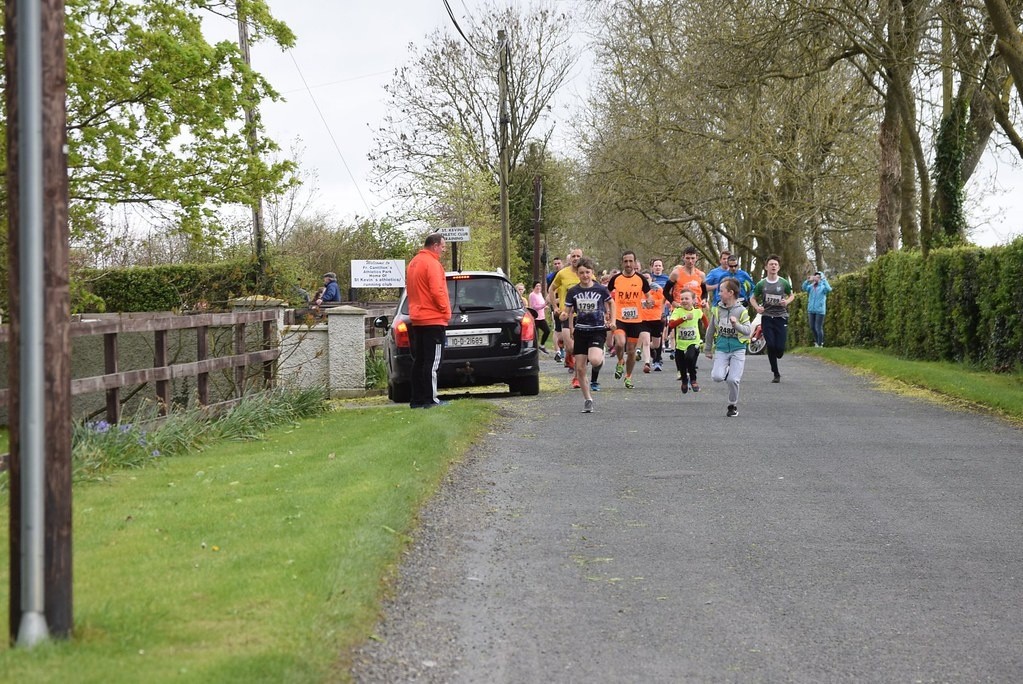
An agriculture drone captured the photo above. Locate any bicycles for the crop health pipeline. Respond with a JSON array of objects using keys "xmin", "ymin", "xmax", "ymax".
[{"xmin": 747, "ymin": 324, "xmax": 767, "ymax": 355}]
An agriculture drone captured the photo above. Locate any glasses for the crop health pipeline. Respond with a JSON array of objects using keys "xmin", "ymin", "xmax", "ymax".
[{"xmin": 728, "ymin": 263, "xmax": 738, "ymax": 268}]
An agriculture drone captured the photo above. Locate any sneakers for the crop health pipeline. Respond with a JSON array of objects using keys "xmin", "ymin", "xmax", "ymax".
[
  {"xmin": 566, "ymin": 353, "xmax": 575, "ymax": 367},
  {"xmin": 727, "ymin": 405, "xmax": 739, "ymax": 417},
  {"xmin": 538, "ymin": 346, "xmax": 549, "ymax": 354},
  {"xmin": 624, "ymin": 377, "xmax": 635, "ymax": 389},
  {"xmin": 590, "ymin": 381, "xmax": 600, "ymax": 391},
  {"xmin": 571, "ymin": 378, "xmax": 580, "ymax": 387},
  {"xmin": 614, "ymin": 361, "xmax": 625, "ymax": 378},
  {"xmin": 583, "ymin": 400, "xmax": 593, "ymax": 413}
]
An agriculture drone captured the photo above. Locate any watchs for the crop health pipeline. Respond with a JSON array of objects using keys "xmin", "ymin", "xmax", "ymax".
[{"xmin": 683, "ymin": 315, "xmax": 687, "ymax": 321}]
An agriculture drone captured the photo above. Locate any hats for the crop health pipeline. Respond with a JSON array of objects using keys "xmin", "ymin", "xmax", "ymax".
[{"xmin": 324, "ymin": 272, "xmax": 338, "ymax": 282}]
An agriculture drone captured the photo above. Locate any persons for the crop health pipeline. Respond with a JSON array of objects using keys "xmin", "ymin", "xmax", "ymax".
[
  {"xmin": 515, "ymin": 281, "xmax": 529, "ymax": 310},
  {"xmin": 291, "ymin": 271, "xmax": 340, "ymax": 307},
  {"xmin": 750, "ymin": 256, "xmax": 794, "ymax": 383},
  {"xmin": 704, "ymin": 278, "xmax": 751, "ymax": 417},
  {"xmin": 546, "ymin": 250, "xmax": 617, "ymax": 414},
  {"xmin": 600, "ymin": 251, "xmax": 655, "ymax": 390},
  {"xmin": 406, "ymin": 234, "xmax": 452, "ymax": 409},
  {"xmin": 801, "ymin": 271, "xmax": 832, "ymax": 347},
  {"xmin": 667, "ymin": 289, "xmax": 707, "ymax": 394},
  {"xmin": 528, "ymin": 280, "xmax": 551, "ymax": 355},
  {"xmin": 704, "ymin": 248, "xmax": 755, "ymax": 341},
  {"xmin": 638, "ymin": 244, "xmax": 707, "ymax": 373}
]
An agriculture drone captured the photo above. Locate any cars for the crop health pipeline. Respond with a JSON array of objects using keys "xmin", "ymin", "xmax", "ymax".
[{"xmin": 373, "ymin": 268, "xmax": 540, "ymax": 403}]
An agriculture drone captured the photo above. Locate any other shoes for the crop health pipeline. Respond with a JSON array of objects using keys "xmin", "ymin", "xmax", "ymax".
[
  {"xmin": 670, "ymin": 354, "xmax": 674, "ymax": 360},
  {"xmin": 815, "ymin": 343, "xmax": 823, "ymax": 348},
  {"xmin": 424, "ymin": 400, "xmax": 451, "ymax": 409},
  {"xmin": 643, "ymin": 348, "xmax": 663, "ymax": 373},
  {"xmin": 686, "ymin": 373, "xmax": 690, "ymax": 379},
  {"xmin": 568, "ymin": 368, "xmax": 574, "ymax": 373},
  {"xmin": 681, "ymin": 381, "xmax": 688, "ymax": 393},
  {"xmin": 608, "ymin": 347, "xmax": 616, "ymax": 357},
  {"xmin": 771, "ymin": 374, "xmax": 780, "ymax": 384},
  {"xmin": 410, "ymin": 401, "xmax": 424, "ymax": 409},
  {"xmin": 554, "ymin": 353, "xmax": 562, "ymax": 363},
  {"xmin": 690, "ymin": 383, "xmax": 699, "ymax": 392},
  {"xmin": 564, "ymin": 361, "xmax": 569, "ymax": 368},
  {"xmin": 676, "ymin": 371, "xmax": 681, "ymax": 380},
  {"xmin": 635, "ymin": 351, "xmax": 642, "ymax": 361}
]
